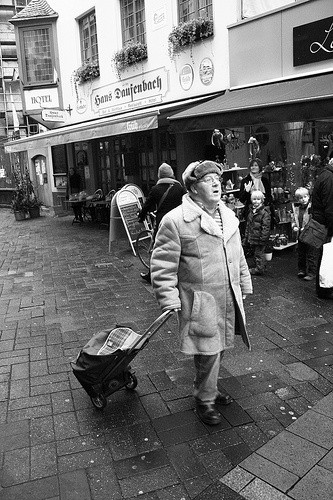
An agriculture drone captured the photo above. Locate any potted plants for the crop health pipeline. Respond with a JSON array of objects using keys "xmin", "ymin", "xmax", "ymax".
[{"xmin": 11, "ymin": 167, "xmax": 44, "ymax": 222}]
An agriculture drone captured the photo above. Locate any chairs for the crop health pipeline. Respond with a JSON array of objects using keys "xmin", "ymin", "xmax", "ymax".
[{"xmin": 83, "ymin": 189, "xmax": 119, "ymax": 226}]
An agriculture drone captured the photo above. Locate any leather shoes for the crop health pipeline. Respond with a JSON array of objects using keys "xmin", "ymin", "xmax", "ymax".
[
  {"xmin": 216, "ymin": 391, "xmax": 230, "ymax": 405},
  {"xmin": 140, "ymin": 272, "xmax": 151, "ymax": 284},
  {"xmin": 194, "ymin": 402, "xmax": 220, "ymax": 424}
]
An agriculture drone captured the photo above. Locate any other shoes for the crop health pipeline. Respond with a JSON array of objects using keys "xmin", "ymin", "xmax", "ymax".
[
  {"xmin": 249, "ymin": 268, "xmax": 259, "ymax": 275},
  {"xmin": 74, "ymin": 217, "xmax": 83, "ymax": 222},
  {"xmin": 317, "ymin": 293, "xmax": 332, "ymax": 299}
]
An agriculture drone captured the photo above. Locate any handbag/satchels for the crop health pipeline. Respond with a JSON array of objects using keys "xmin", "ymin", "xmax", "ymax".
[{"xmin": 299, "ymin": 219, "xmax": 328, "ymax": 249}]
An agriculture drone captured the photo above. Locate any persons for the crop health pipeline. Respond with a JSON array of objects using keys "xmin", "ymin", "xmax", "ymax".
[
  {"xmin": 66, "ymin": 168, "xmax": 85, "ymax": 223},
  {"xmin": 149, "ymin": 160, "xmax": 253, "ymax": 425},
  {"xmin": 291, "ymin": 187, "xmax": 317, "ymax": 280},
  {"xmin": 238, "ymin": 159, "xmax": 276, "ymax": 230},
  {"xmin": 242, "ymin": 190, "xmax": 272, "ymax": 276},
  {"xmin": 137, "ymin": 163, "xmax": 189, "ymax": 284},
  {"xmin": 312, "ymin": 158, "xmax": 333, "ymax": 301}
]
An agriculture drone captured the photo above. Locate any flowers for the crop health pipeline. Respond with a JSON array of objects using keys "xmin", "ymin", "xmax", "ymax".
[{"xmin": 71, "ymin": 17, "xmax": 215, "ymax": 103}]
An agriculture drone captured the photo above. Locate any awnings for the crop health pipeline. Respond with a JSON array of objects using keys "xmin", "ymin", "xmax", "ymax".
[
  {"xmin": 4, "ymin": 91, "xmax": 225, "ymax": 154},
  {"xmin": 167, "ymin": 74, "xmax": 333, "ymax": 134}
]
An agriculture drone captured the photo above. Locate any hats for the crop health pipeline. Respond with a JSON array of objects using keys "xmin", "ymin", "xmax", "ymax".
[
  {"xmin": 158, "ymin": 163, "xmax": 173, "ymax": 178},
  {"xmin": 181, "ymin": 160, "xmax": 223, "ymax": 185}
]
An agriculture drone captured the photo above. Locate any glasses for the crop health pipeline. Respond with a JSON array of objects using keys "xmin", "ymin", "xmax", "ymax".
[{"xmin": 196, "ymin": 176, "xmax": 224, "ymax": 183}]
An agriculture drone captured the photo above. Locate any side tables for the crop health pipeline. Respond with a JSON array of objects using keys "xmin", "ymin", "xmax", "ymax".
[{"xmin": 63, "ymin": 195, "xmax": 100, "ymax": 226}]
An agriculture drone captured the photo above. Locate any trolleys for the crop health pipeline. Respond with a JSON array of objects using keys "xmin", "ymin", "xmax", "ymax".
[{"xmin": 69, "ymin": 308, "xmax": 175, "ymax": 410}]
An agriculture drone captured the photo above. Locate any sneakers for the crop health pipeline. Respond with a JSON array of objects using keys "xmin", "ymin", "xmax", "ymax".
[
  {"xmin": 303, "ymin": 274, "xmax": 316, "ymax": 280},
  {"xmin": 297, "ymin": 270, "xmax": 306, "ymax": 276}
]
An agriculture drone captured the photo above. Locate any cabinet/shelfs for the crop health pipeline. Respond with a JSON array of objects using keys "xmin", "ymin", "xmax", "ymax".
[
  {"xmin": 262, "ymin": 161, "xmax": 298, "ymax": 261},
  {"xmin": 219, "ymin": 166, "xmax": 247, "ymax": 239}
]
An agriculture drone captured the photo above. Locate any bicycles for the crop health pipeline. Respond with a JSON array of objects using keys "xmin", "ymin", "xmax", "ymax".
[{"xmin": 135, "ymin": 211, "xmax": 158, "ymax": 270}]
